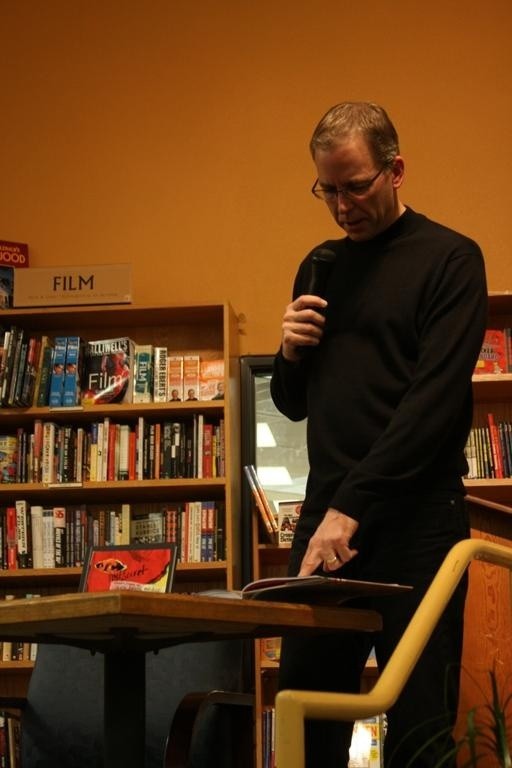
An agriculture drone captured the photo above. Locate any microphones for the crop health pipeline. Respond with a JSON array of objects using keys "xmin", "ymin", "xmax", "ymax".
[{"xmin": 292, "ymin": 248, "xmax": 335, "ymax": 360}]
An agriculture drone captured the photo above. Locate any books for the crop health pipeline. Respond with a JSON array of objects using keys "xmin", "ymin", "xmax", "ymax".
[
  {"xmin": 2, "ymin": 326, "xmax": 226, "ymax": 483},
  {"xmin": 471, "ymin": 325, "xmax": 512, "ymax": 374},
  {"xmin": 3, "ymin": 594, "xmax": 41, "ymax": 661},
  {"xmin": 262, "ymin": 706, "xmax": 383, "ymax": 768},
  {"xmin": 1, "ymin": 712, "xmax": 23, "ymax": 767},
  {"xmin": 245, "ymin": 464, "xmax": 306, "ymax": 543},
  {"xmin": 464, "ymin": 413, "xmax": 512, "ymax": 479},
  {"xmin": 2, "ymin": 501, "xmax": 224, "ymax": 592}
]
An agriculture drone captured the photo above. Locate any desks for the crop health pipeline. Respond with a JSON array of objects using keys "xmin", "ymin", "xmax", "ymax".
[{"xmin": 0, "ymin": 588, "xmax": 391, "ymax": 767}]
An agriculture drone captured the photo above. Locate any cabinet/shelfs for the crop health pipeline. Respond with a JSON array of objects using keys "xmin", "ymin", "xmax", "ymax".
[
  {"xmin": 0, "ymin": 296, "xmax": 243, "ymax": 705},
  {"xmin": 464, "ymin": 286, "xmax": 512, "ymax": 540}
]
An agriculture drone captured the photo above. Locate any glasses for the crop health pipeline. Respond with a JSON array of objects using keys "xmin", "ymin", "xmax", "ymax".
[{"xmin": 311, "ymin": 163, "xmax": 389, "ymax": 201}]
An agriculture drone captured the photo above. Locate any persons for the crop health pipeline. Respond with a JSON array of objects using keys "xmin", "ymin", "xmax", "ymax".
[{"xmin": 269, "ymin": 101, "xmax": 487, "ymax": 768}]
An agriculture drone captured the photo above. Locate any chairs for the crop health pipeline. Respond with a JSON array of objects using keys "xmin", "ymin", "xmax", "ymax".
[{"xmin": 1, "ymin": 581, "xmax": 262, "ymax": 767}]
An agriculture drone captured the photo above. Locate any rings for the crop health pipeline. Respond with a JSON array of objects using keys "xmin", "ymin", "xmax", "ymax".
[{"xmin": 327, "ymin": 556, "xmax": 336, "ymax": 564}]
